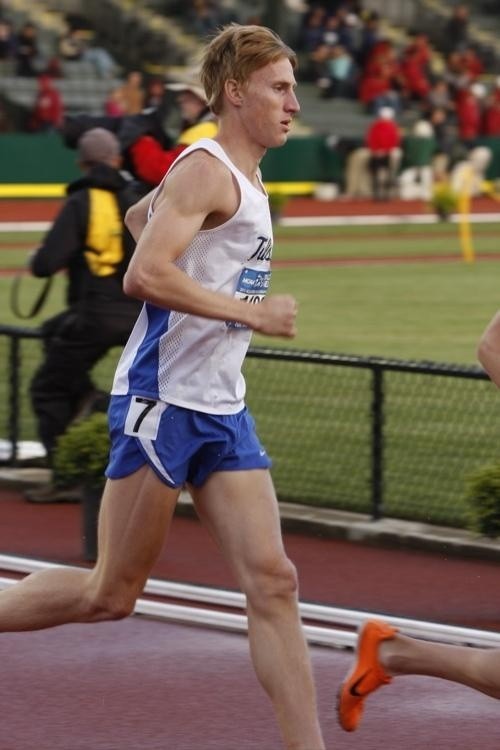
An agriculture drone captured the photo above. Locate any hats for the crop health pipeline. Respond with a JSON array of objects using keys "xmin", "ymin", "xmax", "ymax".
[{"xmin": 165, "ymin": 69, "xmax": 209, "ymax": 106}]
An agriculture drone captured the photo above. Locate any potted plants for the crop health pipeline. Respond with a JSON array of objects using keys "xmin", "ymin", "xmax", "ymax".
[{"xmin": 52, "ymin": 413, "xmax": 117, "ymax": 563}]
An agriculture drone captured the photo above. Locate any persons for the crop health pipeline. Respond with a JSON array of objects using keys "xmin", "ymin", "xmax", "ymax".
[
  {"xmin": 176, "ymin": 1, "xmax": 500, "ymax": 204},
  {"xmin": 337, "ymin": 309, "xmax": 500, "ymax": 731},
  {"xmin": 24, "ymin": 128, "xmax": 146, "ymax": 505},
  {"xmin": 2, "ymin": 22, "xmax": 327, "ymax": 749},
  {"xmin": 0, "ymin": 10, "xmax": 217, "ymax": 181}
]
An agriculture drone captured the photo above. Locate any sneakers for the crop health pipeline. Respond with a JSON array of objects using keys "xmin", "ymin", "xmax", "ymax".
[
  {"xmin": 22, "ymin": 486, "xmax": 85, "ymax": 504},
  {"xmin": 338, "ymin": 620, "xmax": 400, "ymax": 732}
]
